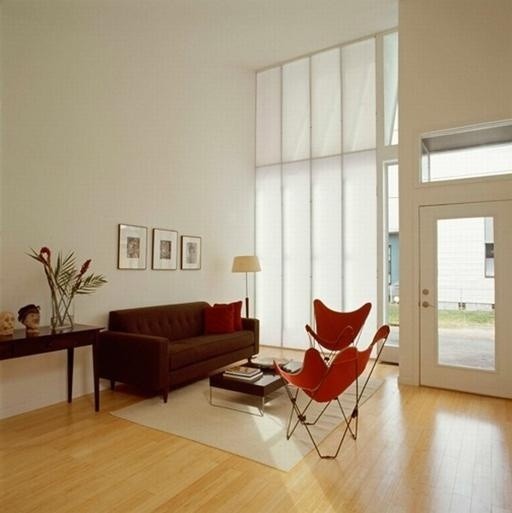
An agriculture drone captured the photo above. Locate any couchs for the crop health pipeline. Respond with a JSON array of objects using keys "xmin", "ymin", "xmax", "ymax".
[{"xmin": 100, "ymin": 302, "xmax": 259, "ymax": 402}]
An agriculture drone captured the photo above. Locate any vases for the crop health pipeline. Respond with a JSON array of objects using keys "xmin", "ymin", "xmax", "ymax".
[{"xmin": 50, "ymin": 295, "xmax": 74, "ymax": 329}]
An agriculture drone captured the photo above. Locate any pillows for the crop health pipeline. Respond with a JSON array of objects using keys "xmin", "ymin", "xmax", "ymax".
[{"xmin": 204, "ymin": 300, "xmax": 242, "ymax": 334}]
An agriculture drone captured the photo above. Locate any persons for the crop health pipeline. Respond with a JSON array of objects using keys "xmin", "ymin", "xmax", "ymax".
[
  {"xmin": 17, "ymin": 303, "xmax": 41, "ymax": 333},
  {"xmin": 1, "ymin": 309, "xmax": 16, "ymax": 335}
]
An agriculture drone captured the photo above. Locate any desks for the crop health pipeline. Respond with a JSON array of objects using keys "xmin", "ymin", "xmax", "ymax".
[{"xmin": 0, "ymin": 322, "xmax": 105, "ymax": 411}]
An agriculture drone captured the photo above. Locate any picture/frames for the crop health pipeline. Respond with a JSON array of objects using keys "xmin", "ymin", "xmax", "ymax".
[{"xmin": 118, "ymin": 224, "xmax": 201, "ymax": 270}]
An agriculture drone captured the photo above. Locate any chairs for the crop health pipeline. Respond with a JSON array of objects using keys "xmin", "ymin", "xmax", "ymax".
[{"xmin": 273, "ymin": 299, "xmax": 390, "ymax": 458}]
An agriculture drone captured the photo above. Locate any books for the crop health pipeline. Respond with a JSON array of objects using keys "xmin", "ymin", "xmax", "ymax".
[
  {"xmin": 223, "ymin": 372, "xmax": 263, "ymax": 383},
  {"xmin": 225, "ymin": 365, "xmax": 262, "ymax": 377}
]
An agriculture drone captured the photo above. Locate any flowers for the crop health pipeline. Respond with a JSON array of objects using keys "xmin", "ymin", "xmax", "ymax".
[{"xmin": 24, "ymin": 246, "xmax": 109, "ymax": 329}]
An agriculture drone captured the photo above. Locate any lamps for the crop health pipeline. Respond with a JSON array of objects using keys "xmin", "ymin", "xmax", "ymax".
[{"xmin": 232, "ymin": 256, "xmax": 262, "ymax": 318}]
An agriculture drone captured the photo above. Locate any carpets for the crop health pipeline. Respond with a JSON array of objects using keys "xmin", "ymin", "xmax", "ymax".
[{"xmin": 109, "ymin": 355, "xmax": 386, "ymax": 473}]
